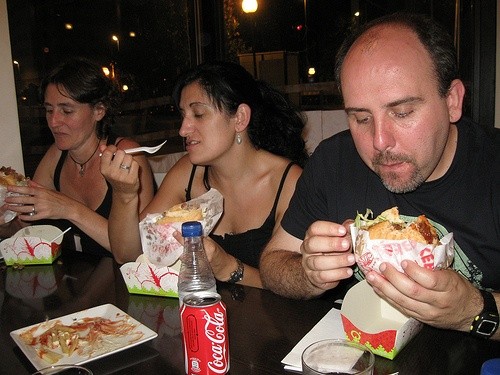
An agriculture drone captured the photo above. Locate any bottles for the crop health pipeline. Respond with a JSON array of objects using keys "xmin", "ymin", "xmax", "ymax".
[{"xmin": 178, "ymin": 221, "xmax": 217, "ymax": 375}]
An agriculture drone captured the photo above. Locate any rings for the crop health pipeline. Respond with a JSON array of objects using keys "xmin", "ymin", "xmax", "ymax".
[
  {"xmin": 120, "ymin": 165, "xmax": 130, "ymax": 170},
  {"xmin": 29, "ymin": 207, "xmax": 35, "ymax": 216}
]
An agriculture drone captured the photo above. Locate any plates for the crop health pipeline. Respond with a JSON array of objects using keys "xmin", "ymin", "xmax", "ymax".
[{"xmin": 10, "ymin": 303, "xmax": 159, "ymax": 374}]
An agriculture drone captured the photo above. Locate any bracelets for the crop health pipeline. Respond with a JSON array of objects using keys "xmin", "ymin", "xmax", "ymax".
[{"xmin": 223, "ymin": 258, "xmax": 244, "ymax": 285}]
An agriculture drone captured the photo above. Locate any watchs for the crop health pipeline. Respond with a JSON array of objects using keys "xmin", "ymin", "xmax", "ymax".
[{"xmin": 469, "ymin": 289, "xmax": 500, "ymax": 340}]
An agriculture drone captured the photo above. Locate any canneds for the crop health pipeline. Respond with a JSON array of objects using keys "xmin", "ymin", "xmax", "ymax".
[{"xmin": 179, "ymin": 291, "xmax": 230, "ymax": 375}]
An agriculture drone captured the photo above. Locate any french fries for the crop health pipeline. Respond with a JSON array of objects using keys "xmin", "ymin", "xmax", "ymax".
[{"xmin": 30, "ymin": 324, "xmax": 79, "ymax": 363}]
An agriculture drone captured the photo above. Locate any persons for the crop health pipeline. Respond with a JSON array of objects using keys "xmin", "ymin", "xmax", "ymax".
[
  {"xmin": 0, "ymin": 59, "xmax": 154, "ymax": 257},
  {"xmin": 99, "ymin": 61, "xmax": 312, "ymax": 290},
  {"xmin": 259, "ymin": 11, "xmax": 500, "ymax": 341}
]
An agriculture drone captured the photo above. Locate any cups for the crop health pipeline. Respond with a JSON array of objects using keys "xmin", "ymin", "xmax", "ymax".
[
  {"xmin": 300, "ymin": 338, "xmax": 376, "ymax": 375},
  {"xmin": 31, "ymin": 364, "xmax": 93, "ymax": 375}
]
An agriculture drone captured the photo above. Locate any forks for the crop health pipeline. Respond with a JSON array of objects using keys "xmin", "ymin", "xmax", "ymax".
[{"xmin": 99, "ymin": 139, "xmax": 167, "ymax": 157}]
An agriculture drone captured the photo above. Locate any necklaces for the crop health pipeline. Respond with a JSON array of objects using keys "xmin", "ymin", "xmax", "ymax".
[{"xmin": 68, "ymin": 135, "xmax": 102, "ymax": 176}]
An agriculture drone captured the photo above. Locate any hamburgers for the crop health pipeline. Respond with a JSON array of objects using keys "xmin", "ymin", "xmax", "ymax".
[
  {"xmin": 354, "ymin": 206, "xmax": 440, "ymax": 244},
  {"xmin": 0, "ymin": 166, "xmax": 28, "ymax": 188},
  {"xmin": 154, "ymin": 202, "xmax": 203, "ymax": 224}
]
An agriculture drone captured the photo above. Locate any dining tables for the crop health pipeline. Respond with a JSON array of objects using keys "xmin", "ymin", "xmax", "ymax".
[{"xmin": 0, "ymin": 238, "xmax": 500, "ymax": 375}]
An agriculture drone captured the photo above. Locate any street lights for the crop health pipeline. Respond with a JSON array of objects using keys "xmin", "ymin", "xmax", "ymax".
[
  {"xmin": 15, "ymin": 23, "xmax": 135, "ymax": 101},
  {"xmin": 241, "ymin": 0, "xmax": 259, "ymax": 81}
]
